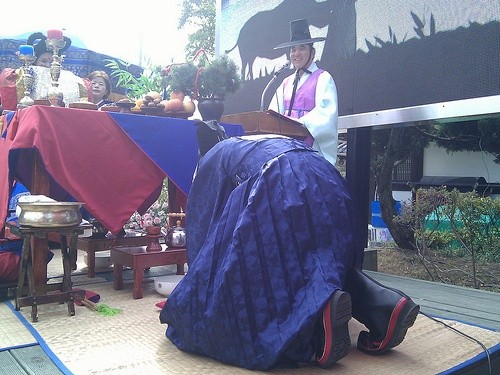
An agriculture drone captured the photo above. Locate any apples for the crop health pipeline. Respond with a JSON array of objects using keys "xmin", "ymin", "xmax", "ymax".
[{"xmin": 147, "ymin": 88, "xmax": 195, "ymax": 114}]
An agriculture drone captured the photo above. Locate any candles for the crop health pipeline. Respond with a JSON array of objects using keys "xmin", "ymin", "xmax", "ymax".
[
  {"xmin": 48, "ymin": 28, "xmax": 63, "ymax": 40},
  {"xmin": 19, "ymin": 44, "xmax": 34, "ymax": 55}
]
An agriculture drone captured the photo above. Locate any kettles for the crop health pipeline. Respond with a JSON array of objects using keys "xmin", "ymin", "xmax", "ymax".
[{"xmin": 164, "ymin": 220, "xmax": 187, "ymax": 249}]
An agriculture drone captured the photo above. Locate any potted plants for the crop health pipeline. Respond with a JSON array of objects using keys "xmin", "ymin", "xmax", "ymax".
[{"xmin": 167, "ymin": 50, "xmax": 242, "ymax": 120}]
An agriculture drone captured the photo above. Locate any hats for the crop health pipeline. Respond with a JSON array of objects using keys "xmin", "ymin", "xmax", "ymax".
[{"xmin": 274, "ymin": 18, "xmax": 327, "ymax": 50}]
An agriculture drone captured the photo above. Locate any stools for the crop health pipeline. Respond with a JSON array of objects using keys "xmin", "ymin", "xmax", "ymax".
[
  {"xmin": 16, "ymin": 224, "xmax": 86, "ymax": 323},
  {"xmin": 69, "ymin": 230, "xmax": 164, "ymax": 278},
  {"xmin": 110, "ymin": 242, "xmax": 191, "ymax": 299}
]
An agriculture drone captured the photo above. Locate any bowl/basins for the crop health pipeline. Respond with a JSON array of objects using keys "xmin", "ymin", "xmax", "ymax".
[
  {"xmin": 199, "ymin": 97, "xmax": 225, "ymax": 122},
  {"xmin": 141, "ymin": 107, "xmax": 164, "ymax": 116},
  {"xmin": 154, "ymin": 275, "xmax": 185, "ymax": 297},
  {"xmin": 82, "ymin": 252, "xmax": 113, "ymax": 269},
  {"xmin": 17, "ymin": 200, "xmax": 86, "ymax": 227}
]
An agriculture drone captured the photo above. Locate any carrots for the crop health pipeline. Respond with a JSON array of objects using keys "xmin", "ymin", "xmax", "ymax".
[{"xmin": 81, "ymin": 298, "xmax": 97, "ymax": 309}]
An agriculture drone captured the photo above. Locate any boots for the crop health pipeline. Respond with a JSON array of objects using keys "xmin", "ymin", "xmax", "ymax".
[
  {"xmin": 344, "ymin": 266, "xmax": 419, "ymax": 355},
  {"xmin": 313, "ymin": 288, "xmax": 355, "ymax": 367}
]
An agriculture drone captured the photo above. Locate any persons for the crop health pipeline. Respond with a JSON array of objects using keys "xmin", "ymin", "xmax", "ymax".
[
  {"xmin": 88, "ymin": 70, "xmax": 116, "ymax": 111},
  {"xmin": 0, "ymin": 181, "xmax": 52, "ymax": 283},
  {"xmin": 161, "ymin": 119, "xmax": 420, "ymax": 371},
  {"xmin": 268, "ymin": 19, "xmax": 339, "ymax": 169},
  {"xmin": 0, "ymin": 32, "xmax": 80, "ymax": 115}
]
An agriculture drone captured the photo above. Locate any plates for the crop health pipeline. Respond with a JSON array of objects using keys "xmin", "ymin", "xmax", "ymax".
[{"xmin": 159, "ymin": 111, "xmax": 194, "ymax": 119}]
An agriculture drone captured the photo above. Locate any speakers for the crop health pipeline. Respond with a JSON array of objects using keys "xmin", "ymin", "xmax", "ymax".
[{"xmin": 441, "ymin": 176, "xmax": 488, "ymax": 194}]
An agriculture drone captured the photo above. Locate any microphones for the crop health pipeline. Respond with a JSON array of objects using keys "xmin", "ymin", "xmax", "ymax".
[{"xmin": 260, "ymin": 59, "xmax": 291, "ymax": 110}]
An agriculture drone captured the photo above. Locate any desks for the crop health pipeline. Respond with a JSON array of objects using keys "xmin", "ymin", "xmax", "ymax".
[{"xmin": 0, "ymin": 107, "xmax": 250, "ymax": 313}]
